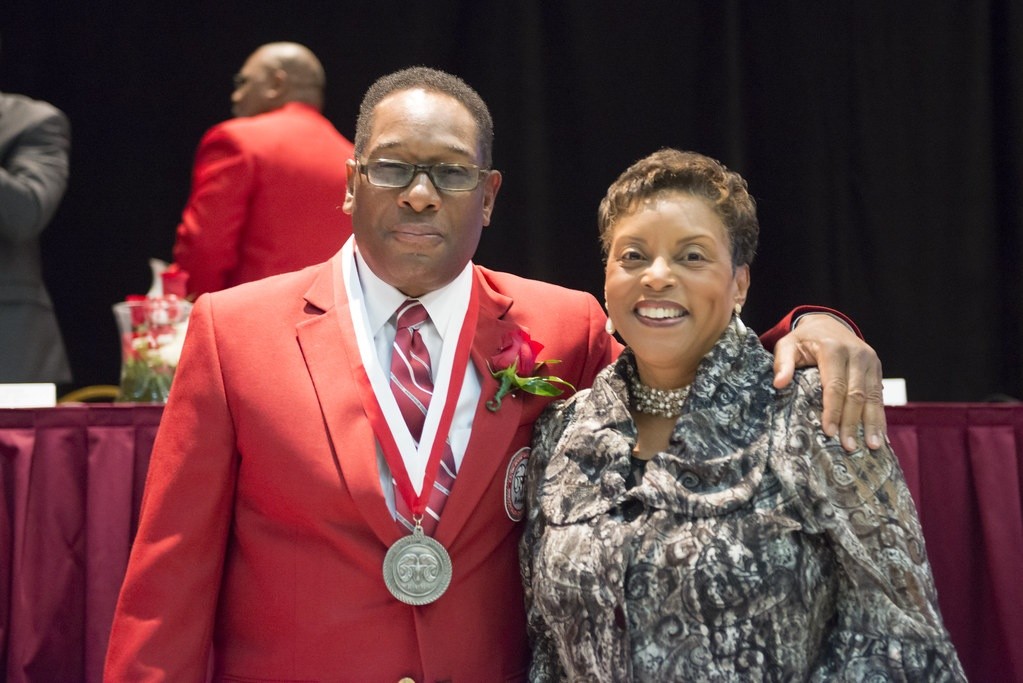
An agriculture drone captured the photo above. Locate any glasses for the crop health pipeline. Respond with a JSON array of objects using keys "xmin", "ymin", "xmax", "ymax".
[{"xmin": 355, "ymin": 156, "xmax": 486, "ymax": 191}]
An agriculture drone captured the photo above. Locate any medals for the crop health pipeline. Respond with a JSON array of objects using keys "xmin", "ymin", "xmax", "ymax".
[{"xmin": 381, "ymin": 526, "xmax": 454, "ymax": 608}]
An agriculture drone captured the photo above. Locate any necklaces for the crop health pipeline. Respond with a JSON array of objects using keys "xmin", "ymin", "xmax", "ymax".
[{"xmin": 629, "ymin": 364, "xmax": 696, "ymax": 418}]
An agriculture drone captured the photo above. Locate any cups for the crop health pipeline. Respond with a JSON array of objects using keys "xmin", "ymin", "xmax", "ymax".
[{"xmin": 112, "ymin": 299, "xmax": 194, "ymax": 402}]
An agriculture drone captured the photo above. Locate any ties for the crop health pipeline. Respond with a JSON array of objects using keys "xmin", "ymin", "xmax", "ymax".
[{"xmin": 388, "ymin": 299, "xmax": 457, "ymax": 539}]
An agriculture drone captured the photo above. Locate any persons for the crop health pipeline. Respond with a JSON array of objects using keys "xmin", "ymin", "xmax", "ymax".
[
  {"xmin": 168, "ymin": 41, "xmax": 361, "ymax": 298},
  {"xmin": 520, "ymin": 150, "xmax": 969, "ymax": 681},
  {"xmin": 0, "ymin": 85, "xmax": 81, "ymax": 407},
  {"xmin": 103, "ymin": 63, "xmax": 891, "ymax": 683}
]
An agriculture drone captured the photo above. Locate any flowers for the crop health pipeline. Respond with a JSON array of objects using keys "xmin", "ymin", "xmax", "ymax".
[{"xmin": 483, "ymin": 331, "xmax": 579, "ymax": 412}]
